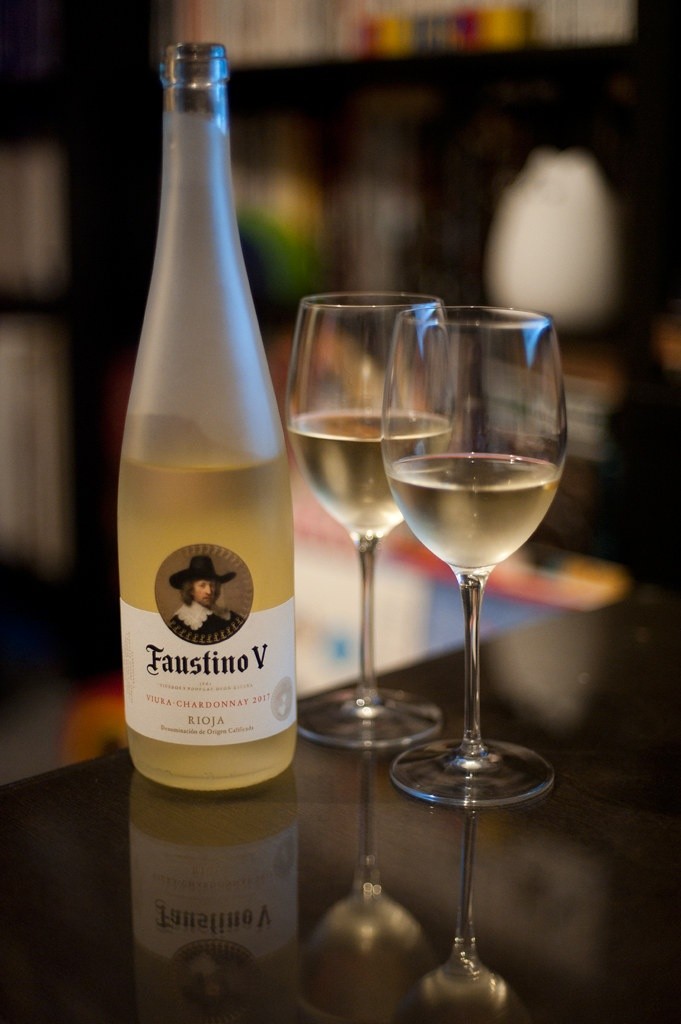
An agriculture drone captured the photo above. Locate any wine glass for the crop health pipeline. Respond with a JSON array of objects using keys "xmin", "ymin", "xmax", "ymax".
[
  {"xmin": 306, "ymin": 755, "xmax": 526, "ymax": 1024},
  {"xmin": 287, "ymin": 292, "xmax": 446, "ymax": 751},
  {"xmin": 383, "ymin": 304, "xmax": 570, "ymax": 805}
]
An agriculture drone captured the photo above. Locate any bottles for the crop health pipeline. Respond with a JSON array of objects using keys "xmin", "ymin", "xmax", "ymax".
[
  {"xmin": 128, "ymin": 771, "xmax": 301, "ymax": 1024},
  {"xmin": 119, "ymin": 42, "xmax": 299, "ymax": 791}
]
